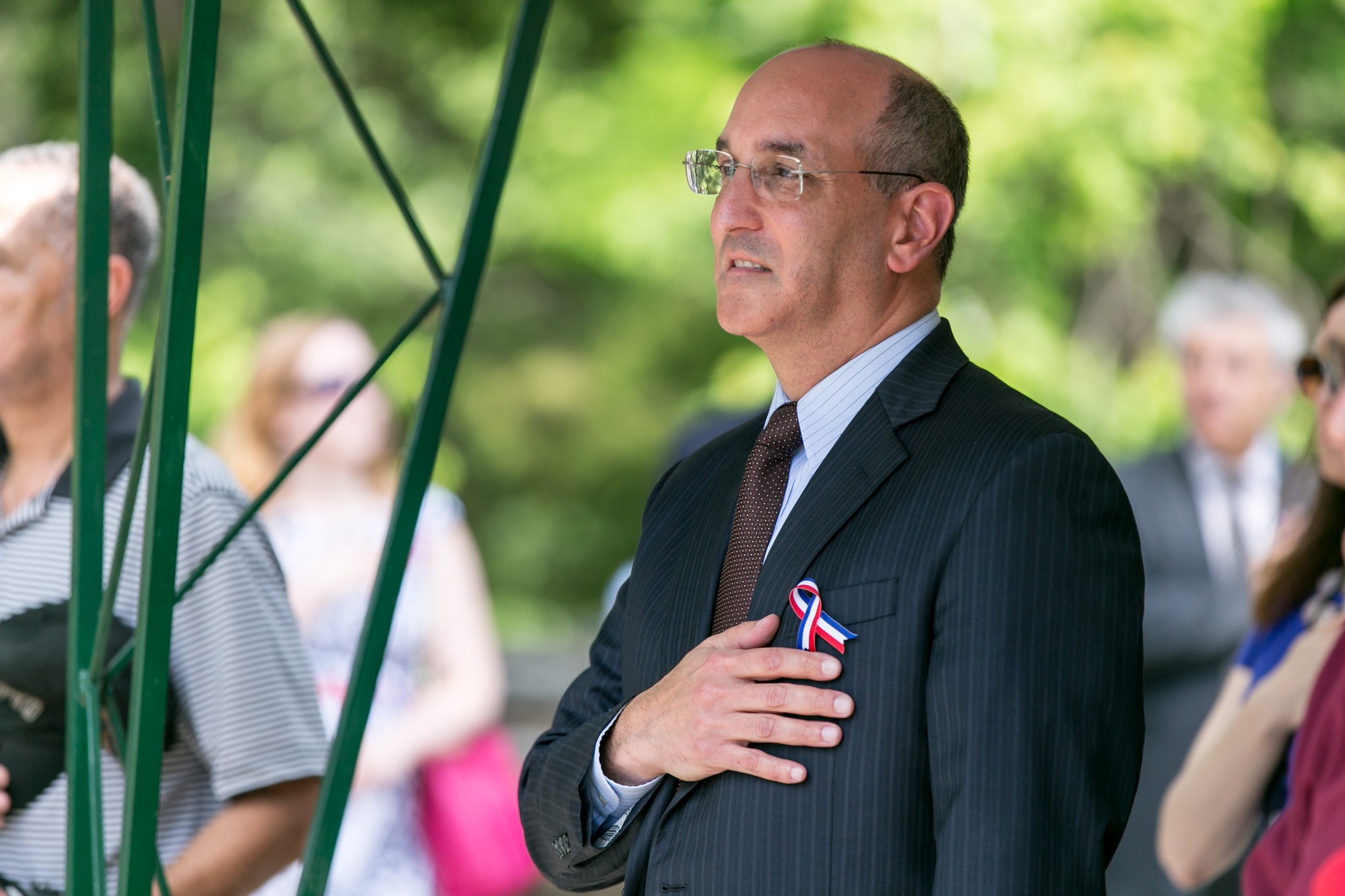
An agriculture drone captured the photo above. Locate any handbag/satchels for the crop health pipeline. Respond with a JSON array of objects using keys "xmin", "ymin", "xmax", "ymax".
[{"xmin": 421, "ymin": 728, "xmax": 542, "ymax": 896}]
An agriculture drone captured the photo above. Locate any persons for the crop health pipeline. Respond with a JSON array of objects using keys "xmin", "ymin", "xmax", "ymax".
[
  {"xmin": 516, "ymin": 43, "xmax": 1345, "ymax": 896},
  {"xmin": 0, "ymin": 139, "xmax": 328, "ymax": 896},
  {"xmin": 212, "ymin": 308, "xmax": 504, "ymax": 895}
]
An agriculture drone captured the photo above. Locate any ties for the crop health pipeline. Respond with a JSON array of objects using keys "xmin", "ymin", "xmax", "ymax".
[
  {"xmin": 709, "ymin": 400, "xmax": 798, "ymax": 637},
  {"xmin": 1226, "ymin": 467, "xmax": 1251, "ymax": 585}
]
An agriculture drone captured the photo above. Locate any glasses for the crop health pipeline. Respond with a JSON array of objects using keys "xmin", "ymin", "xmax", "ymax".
[
  {"xmin": 1296, "ymin": 336, "xmax": 1345, "ymax": 400},
  {"xmin": 682, "ymin": 149, "xmax": 928, "ymax": 201}
]
